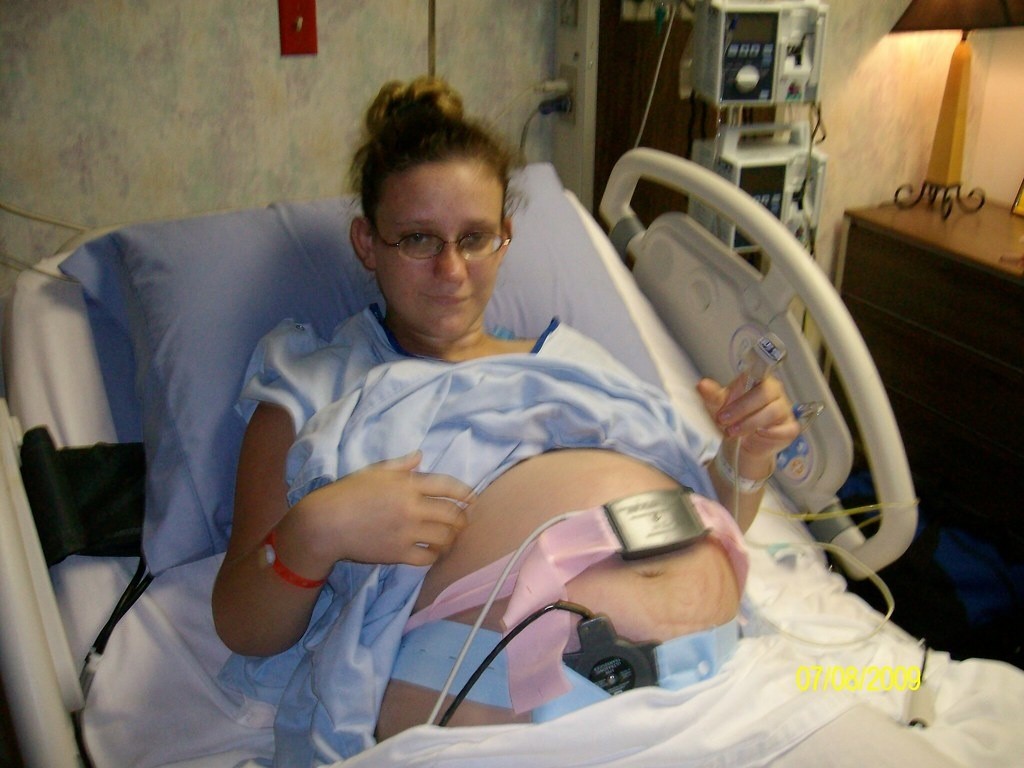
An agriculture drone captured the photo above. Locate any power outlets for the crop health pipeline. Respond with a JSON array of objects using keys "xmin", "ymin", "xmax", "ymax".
[{"xmin": 556, "ymin": 63, "xmax": 579, "ymax": 125}]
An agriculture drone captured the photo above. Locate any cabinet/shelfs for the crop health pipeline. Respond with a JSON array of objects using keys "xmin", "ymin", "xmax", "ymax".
[{"xmin": 827, "ymin": 192, "xmax": 1024, "ymax": 675}]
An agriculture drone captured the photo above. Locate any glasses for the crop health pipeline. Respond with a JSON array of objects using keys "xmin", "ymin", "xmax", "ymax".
[{"xmin": 372, "ymin": 213, "xmax": 511, "ymax": 260}]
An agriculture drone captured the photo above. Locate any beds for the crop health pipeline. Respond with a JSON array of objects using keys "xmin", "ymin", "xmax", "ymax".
[{"xmin": 0, "ymin": 146, "xmax": 1023, "ymax": 768}]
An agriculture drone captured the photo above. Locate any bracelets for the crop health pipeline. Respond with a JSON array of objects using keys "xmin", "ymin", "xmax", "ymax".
[
  {"xmin": 716, "ymin": 446, "xmax": 775, "ymax": 494},
  {"xmin": 264, "ymin": 529, "xmax": 327, "ymax": 588}
]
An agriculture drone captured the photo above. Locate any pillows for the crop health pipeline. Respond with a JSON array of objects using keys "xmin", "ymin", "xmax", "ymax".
[{"xmin": 55, "ymin": 155, "xmax": 686, "ymax": 582}]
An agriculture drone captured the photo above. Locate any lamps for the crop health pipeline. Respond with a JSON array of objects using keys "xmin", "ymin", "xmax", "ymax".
[{"xmin": 889, "ymin": 0, "xmax": 1024, "ymax": 220}]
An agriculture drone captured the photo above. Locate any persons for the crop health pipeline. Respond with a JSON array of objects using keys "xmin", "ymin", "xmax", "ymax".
[{"xmin": 212, "ymin": 77, "xmax": 802, "ymax": 768}]
